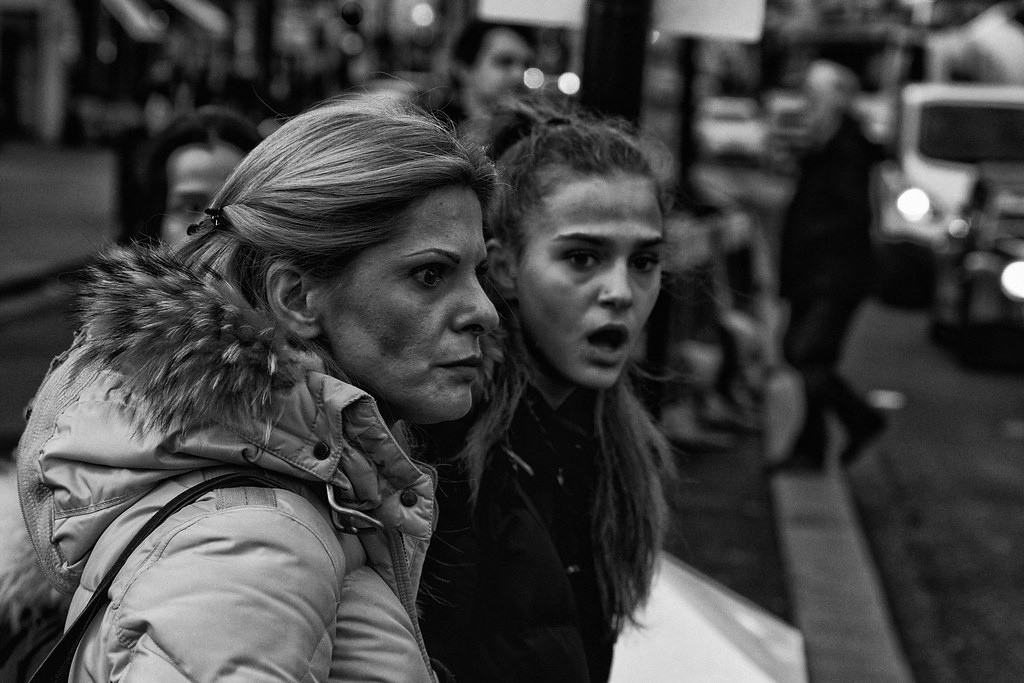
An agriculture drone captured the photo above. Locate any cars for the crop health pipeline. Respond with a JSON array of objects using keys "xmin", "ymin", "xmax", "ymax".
[
  {"xmin": 873, "ymin": 84, "xmax": 1022, "ymax": 300},
  {"xmin": 920, "ymin": 171, "xmax": 1024, "ymax": 363},
  {"xmin": 689, "ymin": 91, "xmax": 763, "ymax": 158}
]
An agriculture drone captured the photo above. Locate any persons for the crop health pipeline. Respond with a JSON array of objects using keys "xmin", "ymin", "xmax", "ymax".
[
  {"xmin": 14, "ymin": 101, "xmax": 500, "ymax": 683},
  {"xmin": 405, "ymin": 117, "xmax": 681, "ymax": 682},
  {"xmin": 446, "ymin": 22, "xmax": 540, "ymax": 157},
  {"xmin": 769, "ymin": 59, "xmax": 885, "ymax": 474},
  {"xmin": 113, "ymin": 88, "xmax": 264, "ymax": 254}
]
